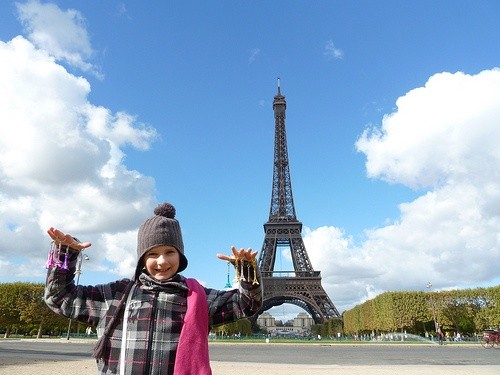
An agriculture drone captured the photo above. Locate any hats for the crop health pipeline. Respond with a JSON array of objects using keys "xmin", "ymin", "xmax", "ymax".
[{"xmin": 92, "ymin": 202, "xmax": 188, "ymax": 360}]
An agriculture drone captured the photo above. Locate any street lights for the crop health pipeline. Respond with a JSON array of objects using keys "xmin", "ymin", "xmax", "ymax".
[
  {"xmin": 67, "ymin": 248, "xmax": 89, "ymax": 341},
  {"xmin": 426, "ymin": 282, "xmax": 437, "ymax": 332}
]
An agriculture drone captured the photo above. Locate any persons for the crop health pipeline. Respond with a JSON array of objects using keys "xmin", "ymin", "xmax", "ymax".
[
  {"xmin": 316, "ymin": 329, "xmax": 464, "ymax": 344},
  {"xmin": 83, "ymin": 325, "xmax": 95, "ymax": 339},
  {"xmin": 208, "ymin": 330, "xmax": 274, "ymax": 340},
  {"xmin": 42, "ymin": 203, "xmax": 263, "ymax": 375}
]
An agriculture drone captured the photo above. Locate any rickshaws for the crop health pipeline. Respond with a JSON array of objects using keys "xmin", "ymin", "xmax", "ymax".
[{"xmin": 481, "ymin": 328, "xmax": 500, "ymax": 349}]
